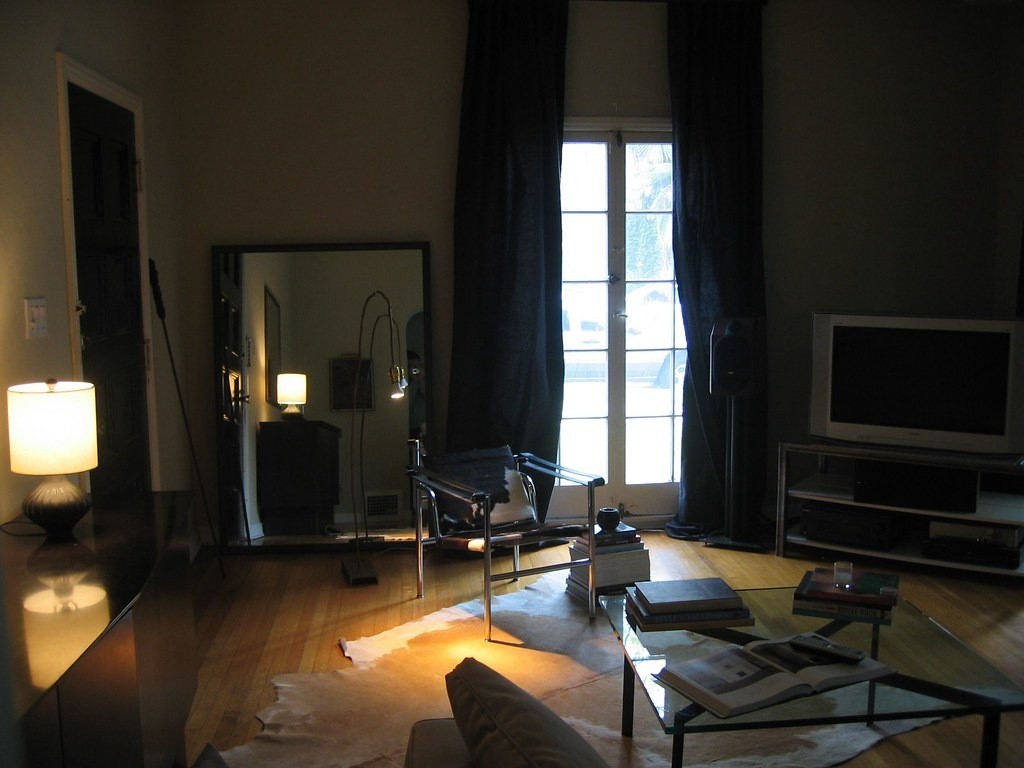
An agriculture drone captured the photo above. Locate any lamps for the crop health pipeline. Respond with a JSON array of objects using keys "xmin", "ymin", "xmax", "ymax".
[
  {"xmin": 350, "ymin": 315, "xmax": 409, "ymax": 542},
  {"xmin": 277, "ymin": 373, "xmax": 306, "ymax": 422},
  {"xmin": 7, "ymin": 379, "xmax": 99, "ymax": 536},
  {"xmin": 342, "ymin": 290, "xmax": 404, "ymax": 586}
]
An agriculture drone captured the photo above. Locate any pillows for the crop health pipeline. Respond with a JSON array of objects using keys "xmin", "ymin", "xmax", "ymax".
[{"xmin": 445, "ymin": 656, "xmax": 609, "ymax": 768}]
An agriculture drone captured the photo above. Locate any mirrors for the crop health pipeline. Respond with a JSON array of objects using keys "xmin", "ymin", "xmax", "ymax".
[
  {"xmin": 407, "ymin": 310, "xmax": 427, "ymax": 511},
  {"xmin": 211, "ymin": 241, "xmax": 435, "ymax": 555}
]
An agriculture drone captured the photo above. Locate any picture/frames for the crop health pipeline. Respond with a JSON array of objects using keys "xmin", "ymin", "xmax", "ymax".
[
  {"xmin": 329, "ymin": 358, "xmax": 376, "ymax": 412},
  {"xmin": 264, "ymin": 285, "xmax": 283, "ymax": 409}
]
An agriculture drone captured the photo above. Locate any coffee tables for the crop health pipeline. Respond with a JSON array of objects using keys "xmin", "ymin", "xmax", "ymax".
[{"xmin": 599, "ymin": 587, "xmax": 1024, "ymax": 768}]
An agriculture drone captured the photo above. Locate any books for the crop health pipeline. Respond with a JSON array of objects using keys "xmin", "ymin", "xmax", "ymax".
[{"xmin": 566, "ymin": 522, "xmax": 899, "ymax": 719}]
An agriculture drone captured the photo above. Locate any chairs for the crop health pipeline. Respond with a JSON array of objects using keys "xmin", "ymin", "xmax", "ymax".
[{"xmin": 406, "ymin": 439, "xmax": 605, "ymax": 642}]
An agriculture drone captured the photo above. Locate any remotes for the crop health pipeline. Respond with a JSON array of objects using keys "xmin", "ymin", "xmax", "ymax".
[{"xmin": 788, "ymin": 635, "xmax": 865, "ymax": 664}]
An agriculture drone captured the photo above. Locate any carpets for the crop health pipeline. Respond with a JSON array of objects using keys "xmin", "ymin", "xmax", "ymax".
[{"xmin": 194, "ymin": 569, "xmax": 960, "ymax": 768}]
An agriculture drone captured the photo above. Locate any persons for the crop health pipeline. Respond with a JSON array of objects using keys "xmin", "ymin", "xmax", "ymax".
[{"xmin": 408, "ymin": 350, "xmax": 426, "ymax": 441}]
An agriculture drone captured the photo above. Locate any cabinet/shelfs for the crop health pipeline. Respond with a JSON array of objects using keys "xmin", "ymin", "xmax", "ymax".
[
  {"xmin": 775, "ymin": 441, "xmax": 1024, "ymax": 579},
  {"xmin": 0, "ymin": 490, "xmax": 198, "ymax": 768},
  {"xmin": 259, "ymin": 420, "xmax": 343, "ymax": 536}
]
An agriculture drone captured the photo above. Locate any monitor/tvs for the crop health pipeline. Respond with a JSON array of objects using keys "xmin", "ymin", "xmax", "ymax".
[{"xmin": 809, "ymin": 312, "xmax": 1024, "ymax": 456}]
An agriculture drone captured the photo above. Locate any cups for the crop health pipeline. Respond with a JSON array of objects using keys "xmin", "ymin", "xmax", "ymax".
[{"xmin": 834, "ymin": 561, "xmax": 852, "ymax": 589}]
